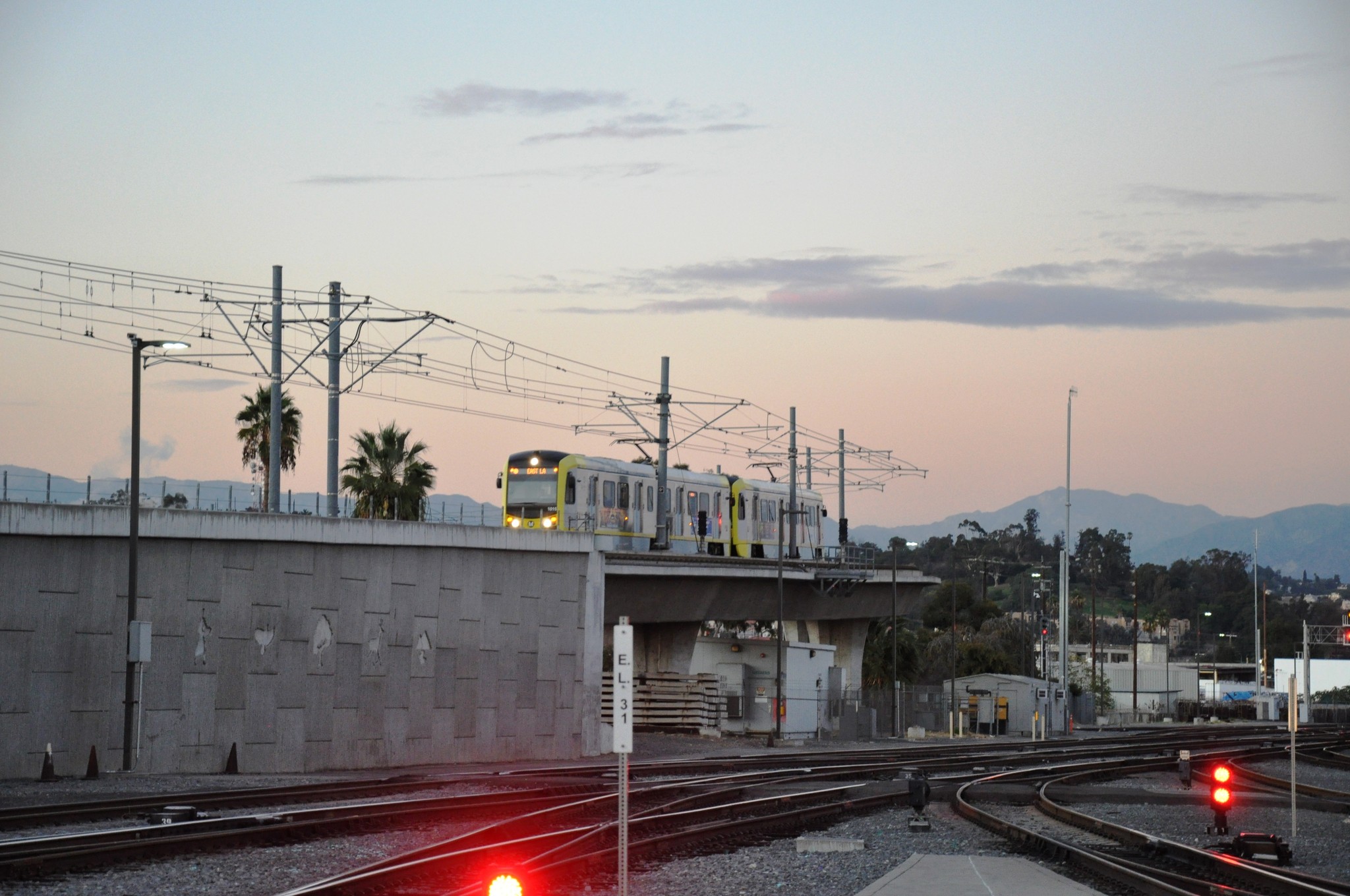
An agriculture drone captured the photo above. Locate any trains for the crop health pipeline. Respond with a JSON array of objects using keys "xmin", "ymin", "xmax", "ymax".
[{"xmin": 496, "ymin": 449, "xmax": 828, "ymax": 564}]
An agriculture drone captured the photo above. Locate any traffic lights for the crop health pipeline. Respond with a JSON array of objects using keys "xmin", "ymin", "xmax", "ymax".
[
  {"xmin": 1208, "ymin": 764, "xmax": 1233, "ymax": 813},
  {"xmin": 485, "ymin": 863, "xmax": 530, "ymax": 896}
]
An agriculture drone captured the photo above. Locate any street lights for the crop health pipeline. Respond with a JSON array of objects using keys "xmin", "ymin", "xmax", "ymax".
[
  {"xmin": 891, "ymin": 540, "xmax": 919, "ymax": 742},
  {"xmin": 1020, "ymin": 571, "xmax": 1041, "ymax": 676},
  {"xmin": 1063, "ymin": 386, "xmax": 1079, "ymax": 690},
  {"xmin": 1210, "ymin": 633, "xmax": 1226, "ymax": 723},
  {"xmin": 1193, "ymin": 610, "xmax": 1212, "ymax": 727},
  {"xmin": 952, "ymin": 554, "xmax": 979, "ymax": 739},
  {"xmin": 115, "ymin": 333, "xmax": 192, "ymax": 777}
]
